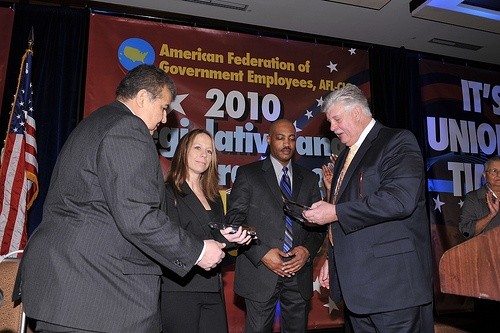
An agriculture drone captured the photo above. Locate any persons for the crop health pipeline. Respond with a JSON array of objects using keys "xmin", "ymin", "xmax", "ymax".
[
  {"xmin": 21, "ymin": 64, "xmax": 226, "ymax": 333},
  {"xmin": 160, "ymin": 129, "xmax": 257, "ymax": 333},
  {"xmin": 303, "ymin": 84, "xmax": 433, "ymax": 333},
  {"xmin": 457, "ymin": 155, "xmax": 500, "ymax": 240},
  {"xmin": 226, "ymin": 119, "xmax": 327, "ymax": 333}
]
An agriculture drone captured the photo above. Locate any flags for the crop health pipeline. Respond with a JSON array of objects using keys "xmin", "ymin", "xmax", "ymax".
[{"xmin": 0, "ymin": 51, "xmax": 39, "ymax": 262}]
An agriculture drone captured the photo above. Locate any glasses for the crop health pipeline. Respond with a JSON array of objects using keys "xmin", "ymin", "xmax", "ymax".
[{"xmin": 485, "ymin": 168, "xmax": 500, "ymax": 175}]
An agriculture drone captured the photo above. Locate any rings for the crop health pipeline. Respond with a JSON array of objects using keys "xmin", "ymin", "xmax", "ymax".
[{"xmin": 311, "ymin": 220, "xmax": 314, "ymax": 223}]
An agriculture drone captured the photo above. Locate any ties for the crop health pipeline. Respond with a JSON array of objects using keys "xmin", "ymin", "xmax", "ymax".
[
  {"xmin": 280, "ymin": 166, "xmax": 294, "ymax": 253},
  {"xmin": 329, "ymin": 144, "xmax": 359, "ymax": 246}
]
